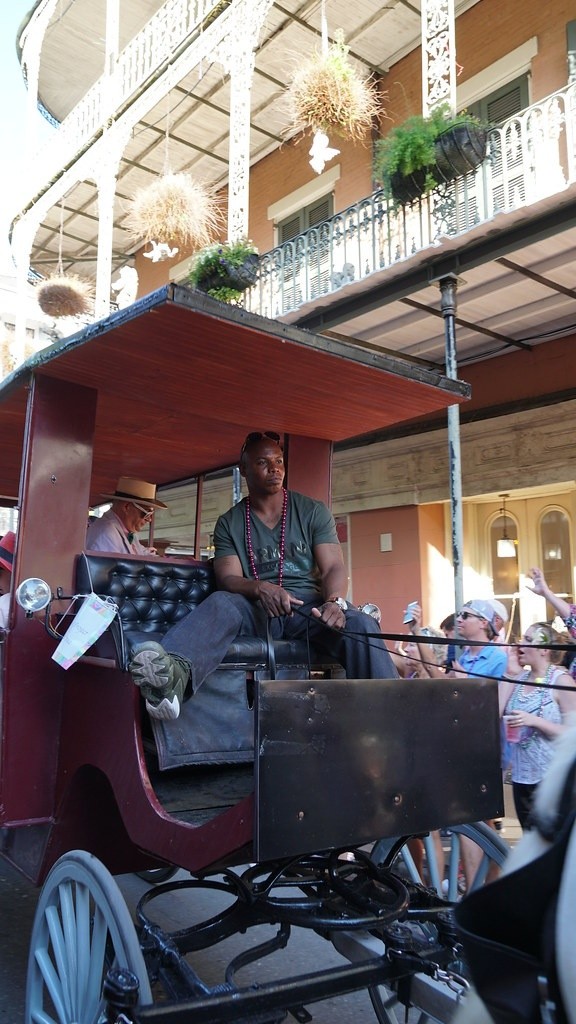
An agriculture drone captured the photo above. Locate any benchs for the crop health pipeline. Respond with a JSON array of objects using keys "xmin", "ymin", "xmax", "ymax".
[{"xmin": 74, "ymin": 553, "xmax": 350, "ymax": 673}]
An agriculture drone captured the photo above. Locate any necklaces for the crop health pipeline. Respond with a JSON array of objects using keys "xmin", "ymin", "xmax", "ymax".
[{"xmin": 246, "ymin": 487, "xmax": 287, "ymax": 590}]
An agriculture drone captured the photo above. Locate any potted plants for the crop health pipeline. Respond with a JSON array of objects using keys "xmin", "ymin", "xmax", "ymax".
[
  {"xmin": 189, "ymin": 236, "xmax": 260, "ymax": 304},
  {"xmin": 371, "ymin": 101, "xmax": 489, "ymax": 219},
  {"xmin": 34, "ymin": 271, "xmax": 90, "ymax": 317}
]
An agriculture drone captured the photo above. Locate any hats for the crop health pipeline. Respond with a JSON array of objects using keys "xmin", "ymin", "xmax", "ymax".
[
  {"xmin": 484, "ymin": 598, "xmax": 508, "ymax": 623},
  {"xmin": 99, "ymin": 476, "xmax": 168, "ymax": 510},
  {"xmin": 463, "ymin": 598, "xmax": 499, "ymax": 636}
]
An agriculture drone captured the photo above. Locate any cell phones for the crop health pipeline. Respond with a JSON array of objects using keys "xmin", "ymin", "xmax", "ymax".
[{"xmin": 403, "ymin": 601, "xmax": 419, "ymax": 624}]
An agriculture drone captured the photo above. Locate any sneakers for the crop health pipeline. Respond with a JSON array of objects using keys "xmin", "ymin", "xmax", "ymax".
[{"xmin": 128, "ymin": 640, "xmax": 194, "ymax": 721}]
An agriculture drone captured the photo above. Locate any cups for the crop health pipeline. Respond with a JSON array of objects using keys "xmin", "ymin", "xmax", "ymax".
[{"xmin": 502, "ymin": 715, "xmax": 521, "ymax": 742}]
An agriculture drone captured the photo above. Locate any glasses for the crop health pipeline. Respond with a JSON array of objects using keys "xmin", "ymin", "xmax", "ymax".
[
  {"xmin": 132, "ymin": 502, "xmax": 155, "ymax": 520},
  {"xmin": 420, "ymin": 628, "xmax": 433, "ymax": 649},
  {"xmin": 456, "ymin": 611, "xmax": 484, "ymax": 620}
]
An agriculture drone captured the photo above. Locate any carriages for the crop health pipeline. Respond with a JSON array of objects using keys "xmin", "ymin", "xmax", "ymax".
[{"xmin": 0, "ymin": 271, "xmax": 575, "ymax": 1024}]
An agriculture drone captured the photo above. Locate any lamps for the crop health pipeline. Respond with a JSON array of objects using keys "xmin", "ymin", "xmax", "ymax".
[
  {"xmin": 496, "ymin": 492, "xmax": 517, "ymax": 558},
  {"xmin": 543, "ymin": 513, "xmax": 561, "ymax": 561}
]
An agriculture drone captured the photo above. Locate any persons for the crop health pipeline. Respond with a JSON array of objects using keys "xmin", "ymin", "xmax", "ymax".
[
  {"xmin": 131, "ymin": 430, "xmax": 402, "ymax": 722},
  {"xmin": 84, "ymin": 475, "xmax": 170, "ymax": 556},
  {"xmin": 1, "ymin": 530, "xmax": 17, "ymax": 635},
  {"xmin": 388, "ymin": 568, "xmax": 575, "ymax": 902}
]
